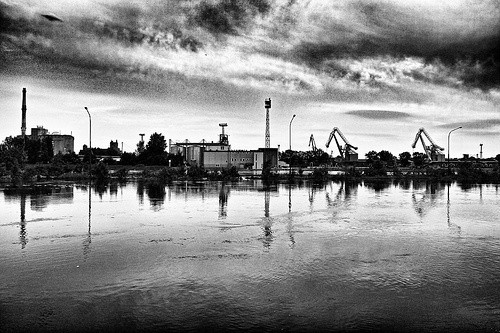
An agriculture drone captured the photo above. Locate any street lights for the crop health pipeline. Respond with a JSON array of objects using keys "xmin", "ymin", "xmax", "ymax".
[
  {"xmin": 84, "ymin": 106, "xmax": 92, "ymax": 169},
  {"xmin": 447, "ymin": 127, "xmax": 463, "ymax": 163},
  {"xmin": 289, "ymin": 114, "xmax": 296, "ymax": 152}
]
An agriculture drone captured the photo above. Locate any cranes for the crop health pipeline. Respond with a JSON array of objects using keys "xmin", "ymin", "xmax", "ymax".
[
  {"xmin": 309, "ymin": 134, "xmax": 317, "ymax": 151},
  {"xmin": 412, "ymin": 127, "xmax": 444, "ymax": 162},
  {"xmin": 326, "ymin": 128, "xmax": 359, "ymax": 161}
]
